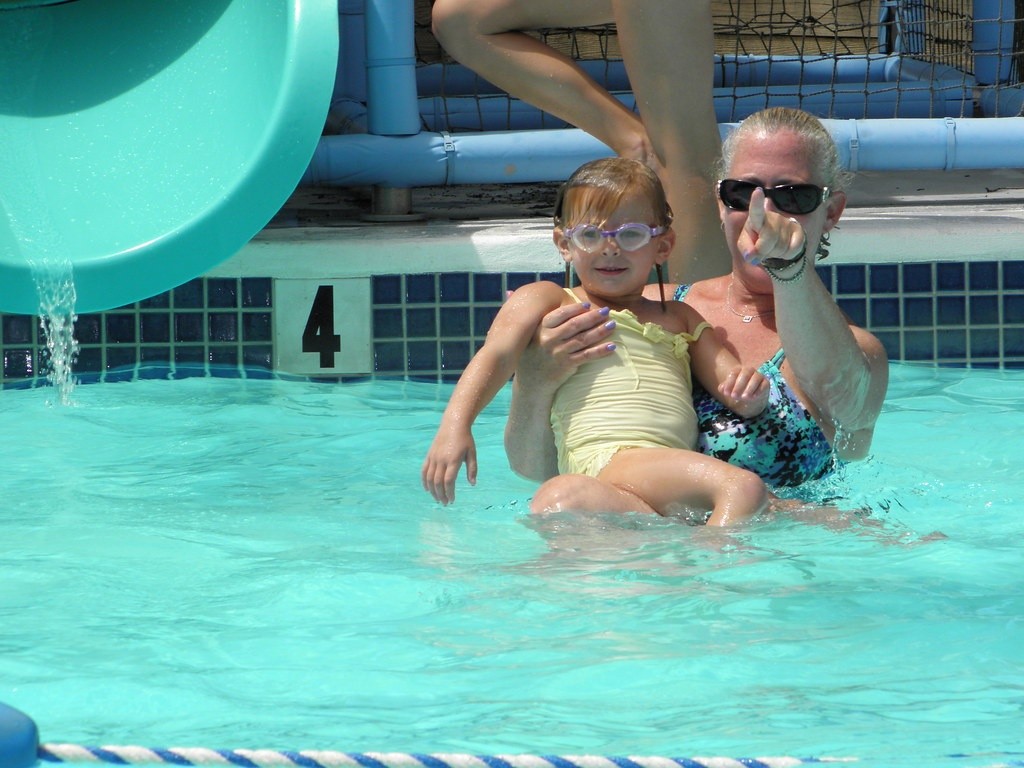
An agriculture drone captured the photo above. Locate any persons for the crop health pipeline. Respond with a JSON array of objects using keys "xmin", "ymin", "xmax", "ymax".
[
  {"xmin": 420, "ymin": 156, "xmax": 950, "ymax": 549},
  {"xmin": 502, "ymin": 105, "xmax": 890, "ymax": 548},
  {"xmin": 431, "ymin": 0, "xmax": 732, "ymax": 287}
]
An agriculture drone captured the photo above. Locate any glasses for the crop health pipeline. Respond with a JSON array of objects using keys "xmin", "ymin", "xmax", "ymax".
[
  {"xmin": 563, "ymin": 223, "xmax": 666, "ymax": 253},
  {"xmin": 716, "ymin": 179, "xmax": 831, "ymax": 216}
]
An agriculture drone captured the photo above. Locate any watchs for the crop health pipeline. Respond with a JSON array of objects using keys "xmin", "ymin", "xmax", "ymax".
[{"xmin": 766, "ymin": 241, "xmax": 808, "ymax": 271}]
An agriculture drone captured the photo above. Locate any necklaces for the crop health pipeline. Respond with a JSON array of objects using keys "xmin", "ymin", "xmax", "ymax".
[{"xmin": 727, "ymin": 270, "xmax": 774, "ymax": 323}]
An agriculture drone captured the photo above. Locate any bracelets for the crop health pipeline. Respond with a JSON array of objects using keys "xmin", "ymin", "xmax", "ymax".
[{"xmin": 765, "ymin": 251, "xmax": 807, "ymax": 283}]
